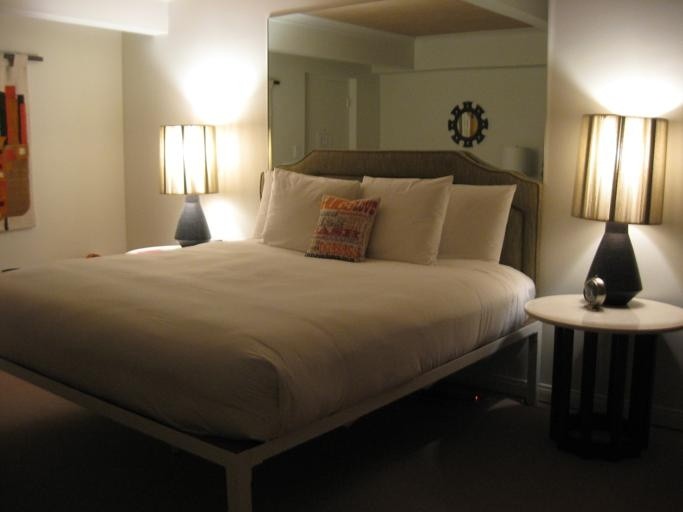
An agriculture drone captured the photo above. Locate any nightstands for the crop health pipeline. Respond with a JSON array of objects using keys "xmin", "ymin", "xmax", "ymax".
[
  {"xmin": 123, "ymin": 243, "xmax": 182, "ymax": 255},
  {"xmin": 522, "ymin": 293, "xmax": 683, "ymax": 461}
]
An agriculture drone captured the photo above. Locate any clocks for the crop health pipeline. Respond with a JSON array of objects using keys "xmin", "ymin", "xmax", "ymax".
[{"xmin": 583, "ymin": 274, "xmax": 608, "ymax": 310}]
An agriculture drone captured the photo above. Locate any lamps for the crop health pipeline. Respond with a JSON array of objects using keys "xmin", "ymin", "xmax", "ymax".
[
  {"xmin": 570, "ymin": 113, "xmax": 669, "ymax": 306},
  {"xmin": 500, "ymin": 144, "xmax": 534, "ymax": 177},
  {"xmin": 158, "ymin": 123, "xmax": 220, "ymax": 249}
]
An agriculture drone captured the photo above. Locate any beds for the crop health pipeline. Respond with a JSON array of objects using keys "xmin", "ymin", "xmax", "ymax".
[{"xmin": 0, "ymin": 147, "xmax": 543, "ymax": 512}]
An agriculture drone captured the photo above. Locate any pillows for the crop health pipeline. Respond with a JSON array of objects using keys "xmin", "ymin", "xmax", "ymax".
[
  {"xmin": 248, "ymin": 167, "xmax": 273, "ymax": 240},
  {"xmin": 362, "ymin": 174, "xmax": 454, "ymax": 267},
  {"xmin": 302, "ymin": 191, "xmax": 382, "ymax": 263},
  {"xmin": 437, "ymin": 183, "xmax": 518, "ymax": 265},
  {"xmin": 260, "ymin": 166, "xmax": 361, "ymax": 252}
]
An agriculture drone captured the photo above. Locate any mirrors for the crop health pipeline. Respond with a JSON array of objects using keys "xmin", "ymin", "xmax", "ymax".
[
  {"xmin": 266, "ymin": 0, "xmax": 550, "ymax": 194},
  {"xmin": 447, "ymin": 100, "xmax": 489, "ymax": 148}
]
